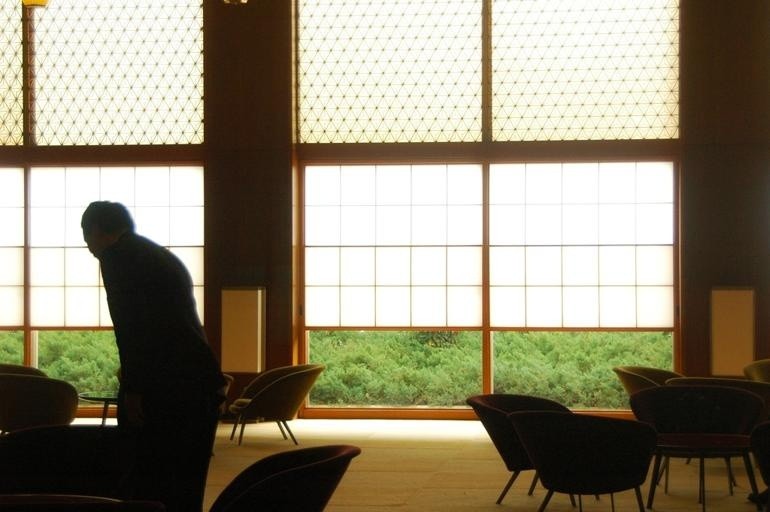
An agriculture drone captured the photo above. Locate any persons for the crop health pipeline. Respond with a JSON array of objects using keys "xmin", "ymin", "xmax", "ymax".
[{"xmin": 79, "ymin": 200, "xmax": 229, "ymax": 511}]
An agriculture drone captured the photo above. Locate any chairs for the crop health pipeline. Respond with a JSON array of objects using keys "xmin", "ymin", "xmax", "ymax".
[
  {"xmin": 466, "ymin": 394, "xmax": 581, "ymax": 505},
  {"xmin": 209, "ymin": 444, "xmax": 361, "ymax": 512},
  {"xmin": 0, "ymin": 363, "xmax": 78, "ymax": 434},
  {"xmin": 743, "ymin": 362, "xmax": 770, "ymax": 474},
  {"xmin": 509, "ymin": 411, "xmax": 651, "ymax": 512},
  {"xmin": 613, "ymin": 365, "xmax": 736, "ymax": 485},
  {"xmin": 666, "ymin": 375, "xmax": 770, "ymax": 505},
  {"xmin": 629, "ymin": 387, "xmax": 759, "ymax": 510},
  {"xmin": 230, "ymin": 364, "xmax": 322, "ymax": 444}
]
